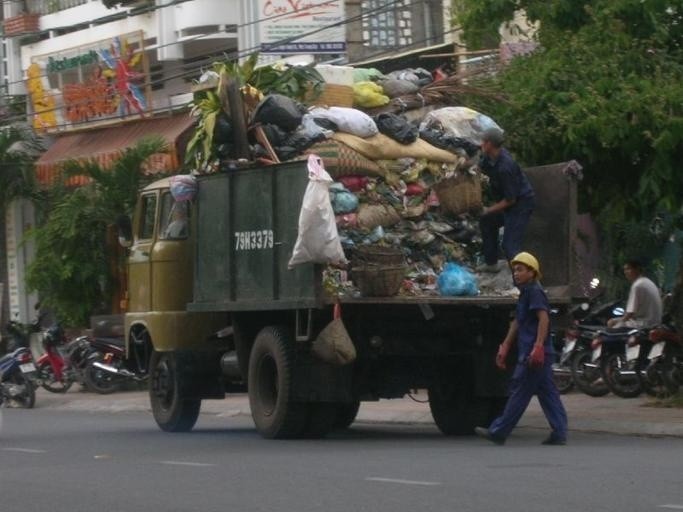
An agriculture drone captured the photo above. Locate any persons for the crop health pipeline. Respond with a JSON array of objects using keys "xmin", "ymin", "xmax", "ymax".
[
  {"xmin": 456, "ymin": 127, "xmax": 537, "ymax": 295},
  {"xmin": 474, "ymin": 252, "xmax": 572, "ymax": 446},
  {"xmin": 605, "ymin": 257, "xmax": 662, "ymax": 329}
]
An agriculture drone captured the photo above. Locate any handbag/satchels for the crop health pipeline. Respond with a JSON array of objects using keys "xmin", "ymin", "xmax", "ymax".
[{"xmin": 290, "ymin": 155, "xmax": 350, "ymax": 267}]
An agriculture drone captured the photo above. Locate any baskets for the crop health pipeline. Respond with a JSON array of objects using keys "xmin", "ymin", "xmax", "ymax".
[
  {"xmin": 349, "ymin": 263, "xmax": 406, "ymax": 297},
  {"xmin": 433, "ymin": 166, "xmax": 483, "ymax": 223},
  {"xmin": 357, "ymin": 244, "xmax": 404, "ymax": 263}
]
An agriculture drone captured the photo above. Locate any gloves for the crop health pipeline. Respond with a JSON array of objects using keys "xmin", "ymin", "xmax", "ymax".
[
  {"xmin": 494, "ymin": 344, "xmax": 509, "ymax": 370},
  {"xmin": 528, "ymin": 345, "xmax": 545, "ymax": 369}
]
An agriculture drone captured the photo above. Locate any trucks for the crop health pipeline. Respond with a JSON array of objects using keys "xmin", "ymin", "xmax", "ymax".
[{"xmin": 124, "ymin": 160, "xmax": 584, "ymax": 440}]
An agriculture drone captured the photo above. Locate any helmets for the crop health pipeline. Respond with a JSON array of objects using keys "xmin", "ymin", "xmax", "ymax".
[{"xmin": 510, "ymin": 251, "xmax": 543, "ymax": 281}]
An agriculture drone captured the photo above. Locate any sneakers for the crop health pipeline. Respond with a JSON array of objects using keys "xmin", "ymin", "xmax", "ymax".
[
  {"xmin": 475, "ymin": 426, "xmax": 505, "ymax": 445},
  {"xmin": 543, "ymin": 434, "xmax": 567, "ymax": 445}
]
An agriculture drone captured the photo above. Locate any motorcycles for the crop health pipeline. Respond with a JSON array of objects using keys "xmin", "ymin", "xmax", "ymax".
[
  {"xmin": 550, "ymin": 278, "xmax": 683, "ymax": 398},
  {"xmin": 0, "ymin": 320, "xmax": 149, "ymax": 408}
]
